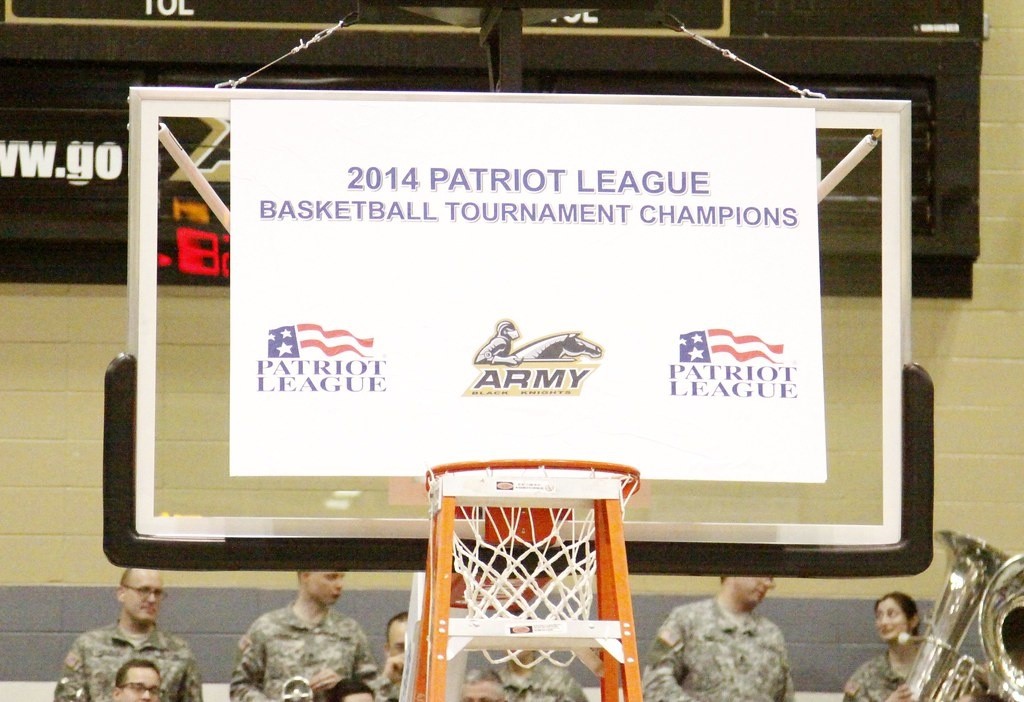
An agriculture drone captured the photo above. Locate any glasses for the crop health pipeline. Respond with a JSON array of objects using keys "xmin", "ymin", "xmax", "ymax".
[
  {"xmin": 123, "ymin": 585, "xmax": 168, "ymax": 600},
  {"xmin": 120, "ymin": 682, "xmax": 165, "ymax": 697}
]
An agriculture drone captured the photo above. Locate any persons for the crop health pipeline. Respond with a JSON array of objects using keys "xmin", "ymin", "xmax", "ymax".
[
  {"xmin": 366, "ymin": 611, "xmax": 408, "ymax": 702},
  {"xmin": 496, "ymin": 609, "xmax": 589, "ymax": 702},
  {"xmin": 843, "ymin": 592, "xmax": 979, "ymax": 702},
  {"xmin": 641, "ymin": 575, "xmax": 797, "ymax": 702},
  {"xmin": 113, "ymin": 658, "xmax": 161, "ymax": 702},
  {"xmin": 54, "ymin": 568, "xmax": 203, "ymax": 702},
  {"xmin": 229, "ymin": 571, "xmax": 383, "ymax": 702},
  {"xmin": 327, "ymin": 678, "xmax": 376, "ymax": 702},
  {"xmin": 460, "ymin": 668, "xmax": 506, "ymax": 702}
]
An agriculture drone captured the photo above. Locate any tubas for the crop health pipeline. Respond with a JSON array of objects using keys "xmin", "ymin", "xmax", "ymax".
[{"xmin": 903, "ymin": 529, "xmax": 1024, "ymax": 702}]
